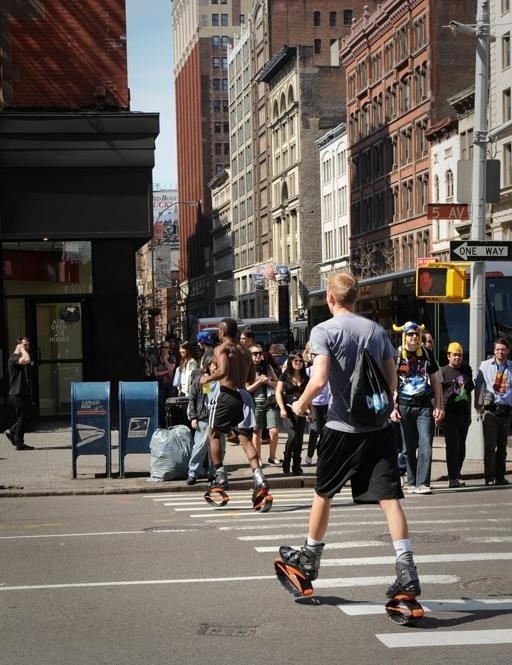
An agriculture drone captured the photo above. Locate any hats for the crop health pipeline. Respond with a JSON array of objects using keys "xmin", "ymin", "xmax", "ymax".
[
  {"xmin": 447, "ymin": 342, "xmax": 463, "ymax": 353},
  {"xmin": 403, "ymin": 321, "xmax": 419, "ymax": 333},
  {"xmin": 196, "ymin": 331, "xmax": 215, "ymax": 344}
]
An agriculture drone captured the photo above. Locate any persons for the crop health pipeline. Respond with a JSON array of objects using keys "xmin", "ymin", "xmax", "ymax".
[
  {"xmin": 4, "ymin": 335, "xmax": 36, "ymax": 451},
  {"xmin": 279, "ymin": 272, "xmax": 422, "ymax": 598}
]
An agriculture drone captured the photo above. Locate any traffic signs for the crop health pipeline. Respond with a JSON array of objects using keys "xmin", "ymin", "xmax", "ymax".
[{"xmin": 449, "ymin": 240, "xmax": 511, "ymax": 263}]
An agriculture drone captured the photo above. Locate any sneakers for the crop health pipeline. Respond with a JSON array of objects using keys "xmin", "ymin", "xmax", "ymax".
[
  {"xmin": 207, "ymin": 467, "xmax": 228, "ymax": 489},
  {"xmin": 449, "ymin": 479, "xmax": 466, "ymax": 488},
  {"xmin": 415, "ymin": 485, "xmax": 432, "ymax": 494},
  {"xmin": 407, "ymin": 486, "xmax": 416, "ymax": 494},
  {"xmin": 186, "ymin": 475, "xmax": 197, "ymax": 486},
  {"xmin": 399, "ymin": 474, "xmax": 404, "ymax": 487},
  {"xmin": 485, "ymin": 481, "xmax": 495, "ymax": 486},
  {"xmin": 5, "ymin": 430, "xmax": 35, "ymax": 450},
  {"xmin": 305, "ymin": 455, "xmax": 312, "ymax": 464},
  {"xmin": 495, "ymin": 479, "xmax": 509, "ymax": 485}
]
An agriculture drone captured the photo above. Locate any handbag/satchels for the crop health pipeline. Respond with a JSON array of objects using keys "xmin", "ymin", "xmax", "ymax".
[{"xmin": 481, "ymin": 392, "xmax": 493, "ymax": 406}]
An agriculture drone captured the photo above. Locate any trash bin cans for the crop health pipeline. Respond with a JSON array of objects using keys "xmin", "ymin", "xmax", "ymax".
[{"xmin": 164, "ymin": 396, "xmax": 196, "ymax": 442}]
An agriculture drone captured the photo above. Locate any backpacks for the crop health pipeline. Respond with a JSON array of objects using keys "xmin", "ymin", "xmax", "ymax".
[{"xmin": 329, "ymin": 318, "xmax": 395, "ymax": 429}]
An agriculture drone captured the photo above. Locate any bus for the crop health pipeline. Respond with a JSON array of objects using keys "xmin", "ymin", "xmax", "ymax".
[
  {"xmin": 289, "ymin": 321, "xmax": 309, "ymax": 350},
  {"xmin": 44, "ymin": 351, "xmax": 81, "ymax": 395},
  {"xmin": 240, "ymin": 318, "xmax": 294, "ymax": 353},
  {"xmin": 307, "ymin": 258, "xmax": 512, "ymax": 378}
]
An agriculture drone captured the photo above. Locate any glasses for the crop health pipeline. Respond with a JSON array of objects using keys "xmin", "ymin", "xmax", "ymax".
[
  {"xmin": 406, "ymin": 332, "xmax": 419, "ymax": 336},
  {"xmin": 292, "ymin": 359, "xmax": 303, "ymax": 363},
  {"xmin": 251, "ymin": 352, "xmax": 262, "ymax": 356}
]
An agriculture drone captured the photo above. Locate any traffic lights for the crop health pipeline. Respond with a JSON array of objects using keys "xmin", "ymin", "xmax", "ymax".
[{"xmin": 414, "ymin": 265, "xmax": 461, "ymax": 300}]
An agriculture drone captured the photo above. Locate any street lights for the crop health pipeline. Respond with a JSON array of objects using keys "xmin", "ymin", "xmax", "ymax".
[{"xmin": 151, "ymin": 199, "xmax": 199, "ymax": 348}]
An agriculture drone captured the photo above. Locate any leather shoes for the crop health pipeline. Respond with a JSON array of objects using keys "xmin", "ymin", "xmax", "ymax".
[
  {"xmin": 259, "ymin": 462, "xmax": 264, "ymax": 468},
  {"xmin": 268, "ymin": 457, "xmax": 283, "ymax": 467}
]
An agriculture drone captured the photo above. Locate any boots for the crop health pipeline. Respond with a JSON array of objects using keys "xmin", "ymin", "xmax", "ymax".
[
  {"xmin": 279, "ymin": 540, "xmax": 325, "ymax": 581},
  {"xmin": 252, "ymin": 468, "xmax": 270, "ymax": 503},
  {"xmin": 385, "ymin": 551, "xmax": 421, "ymax": 599},
  {"xmin": 283, "ymin": 452, "xmax": 303, "ymax": 476}
]
